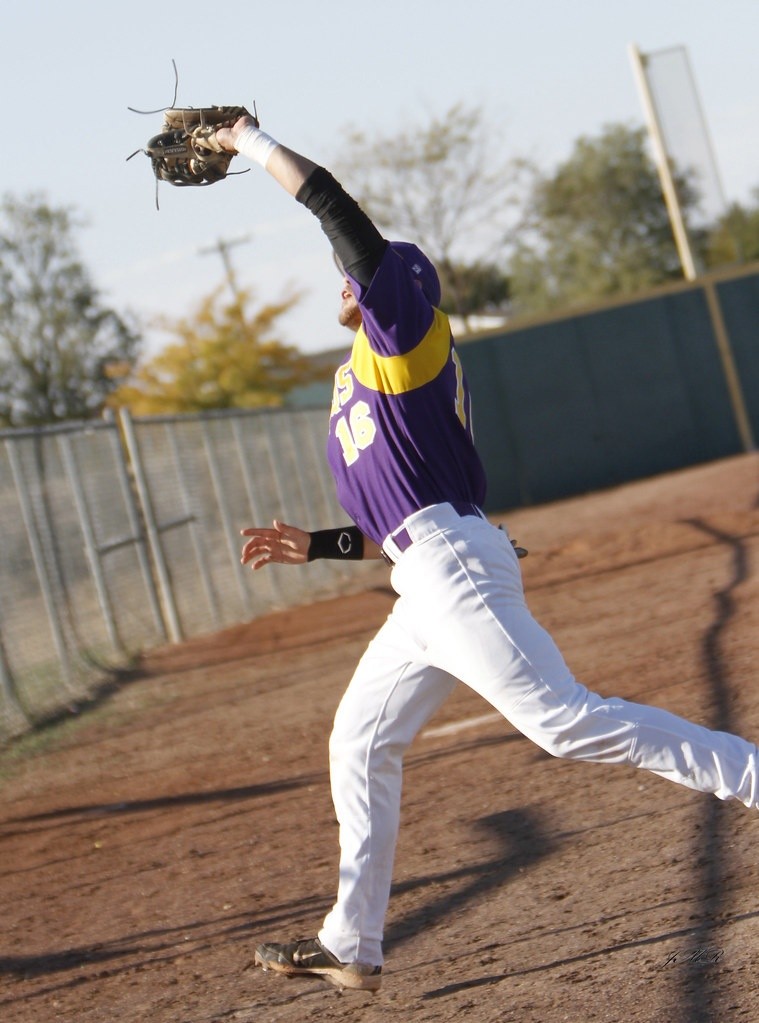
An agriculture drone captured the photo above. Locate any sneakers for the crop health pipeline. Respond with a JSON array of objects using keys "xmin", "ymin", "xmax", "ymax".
[{"xmin": 253, "ymin": 938, "xmax": 383, "ymax": 991}]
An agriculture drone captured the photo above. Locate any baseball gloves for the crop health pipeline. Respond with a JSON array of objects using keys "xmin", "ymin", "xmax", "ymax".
[{"xmin": 149, "ymin": 104, "xmax": 259, "ymax": 188}]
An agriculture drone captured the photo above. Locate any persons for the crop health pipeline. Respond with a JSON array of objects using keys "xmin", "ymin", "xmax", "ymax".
[{"xmin": 194, "ymin": 114, "xmax": 758, "ymax": 992}]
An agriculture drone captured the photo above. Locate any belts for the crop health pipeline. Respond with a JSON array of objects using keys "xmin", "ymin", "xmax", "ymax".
[{"xmin": 381, "ymin": 502, "xmax": 481, "ymax": 565}]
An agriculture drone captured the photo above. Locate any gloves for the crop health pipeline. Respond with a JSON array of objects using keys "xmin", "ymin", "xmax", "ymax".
[{"xmin": 499, "ymin": 523, "xmax": 528, "ymax": 558}]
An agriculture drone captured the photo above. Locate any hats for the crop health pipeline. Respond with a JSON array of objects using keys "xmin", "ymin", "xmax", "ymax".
[{"xmin": 334, "ymin": 242, "xmax": 441, "ymax": 309}]
